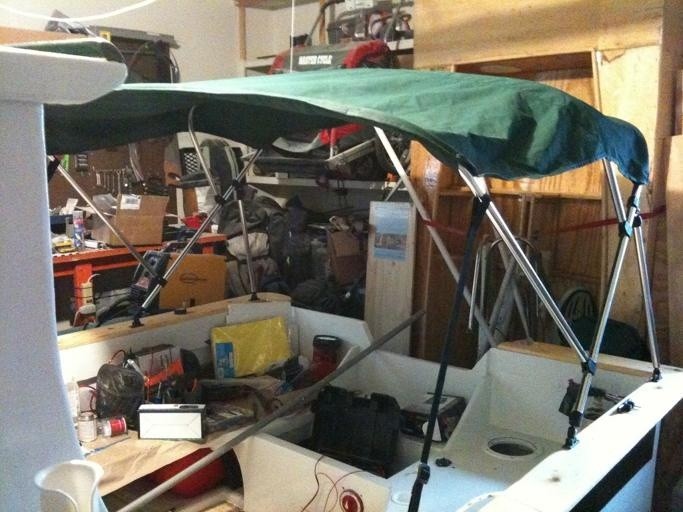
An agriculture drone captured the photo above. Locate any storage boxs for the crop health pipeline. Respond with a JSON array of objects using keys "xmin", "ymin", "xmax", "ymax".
[{"xmin": 91, "ymin": 193, "xmax": 169, "ymax": 248}]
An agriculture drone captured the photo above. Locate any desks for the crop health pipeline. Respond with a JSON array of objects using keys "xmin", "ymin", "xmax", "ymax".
[{"xmin": 54, "ymin": 233, "xmax": 227, "ymax": 326}]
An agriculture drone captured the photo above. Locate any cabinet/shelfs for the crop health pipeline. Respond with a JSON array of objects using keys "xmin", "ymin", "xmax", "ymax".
[
  {"xmin": 48, "ymin": 28, "xmax": 177, "ymax": 217},
  {"xmin": 411, "ymin": 1, "xmax": 673, "ymax": 362},
  {"xmin": 236, "ymin": 1, "xmax": 414, "ymax": 190}
]
